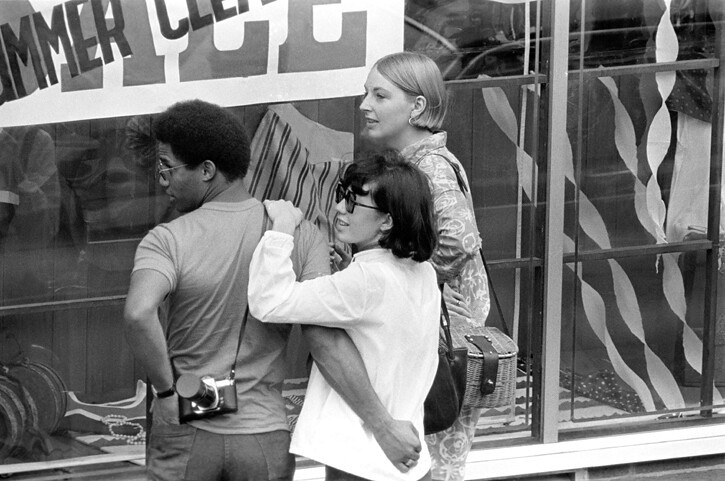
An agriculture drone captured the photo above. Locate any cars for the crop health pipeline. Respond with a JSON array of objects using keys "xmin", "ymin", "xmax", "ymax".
[{"xmin": 405, "ymin": 0, "xmax": 724, "ymax": 81}]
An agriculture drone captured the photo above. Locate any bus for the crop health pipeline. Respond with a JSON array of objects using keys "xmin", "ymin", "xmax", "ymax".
[{"xmin": 0, "ymin": 0, "xmax": 724, "ymax": 481}]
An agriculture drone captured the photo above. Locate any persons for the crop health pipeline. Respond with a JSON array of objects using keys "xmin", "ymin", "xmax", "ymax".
[
  {"xmin": 121, "ymin": 97, "xmax": 422, "ymax": 481},
  {"xmin": 243, "ymin": 51, "xmax": 491, "ymax": 481},
  {"xmin": 0, "ymin": 125, "xmax": 61, "ymax": 257}
]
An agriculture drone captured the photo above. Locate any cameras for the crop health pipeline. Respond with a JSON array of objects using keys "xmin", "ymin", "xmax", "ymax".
[{"xmin": 174, "ymin": 371, "xmax": 238, "ymax": 426}]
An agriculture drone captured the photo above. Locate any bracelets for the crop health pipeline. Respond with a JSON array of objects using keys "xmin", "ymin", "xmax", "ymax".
[{"xmin": 151, "ymin": 384, "xmax": 175, "ymax": 399}]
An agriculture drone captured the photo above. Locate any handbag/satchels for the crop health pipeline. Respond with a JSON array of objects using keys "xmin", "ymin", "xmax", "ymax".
[
  {"xmin": 424, "ymin": 264, "xmax": 468, "ymax": 435},
  {"xmin": 439, "ymin": 327, "xmax": 519, "ymax": 408}
]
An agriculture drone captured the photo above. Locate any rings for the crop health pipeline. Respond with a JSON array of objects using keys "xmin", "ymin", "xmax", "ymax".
[
  {"xmin": 405, "ymin": 459, "xmax": 413, "ymax": 467},
  {"xmin": 456, "ymin": 300, "xmax": 461, "ymax": 306}
]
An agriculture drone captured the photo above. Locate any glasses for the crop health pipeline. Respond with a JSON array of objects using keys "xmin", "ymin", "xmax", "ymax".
[
  {"xmin": 158, "ymin": 159, "xmax": 189, "ymax": 180},
  {"xmin": 336, "ymin": 184, "xmax": 393, "ymax": 217}
]
